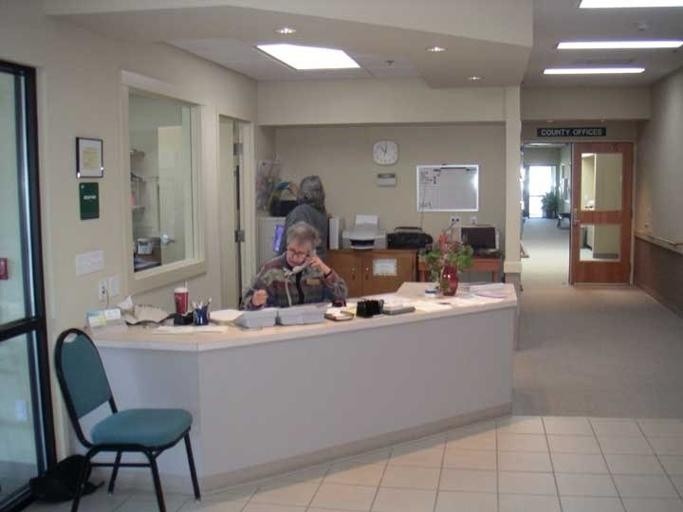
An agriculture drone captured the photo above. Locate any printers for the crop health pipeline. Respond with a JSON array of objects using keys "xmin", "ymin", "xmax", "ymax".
[{"xmin": 342, "ymin": 223, "xmax": 386, "ymax": 249}]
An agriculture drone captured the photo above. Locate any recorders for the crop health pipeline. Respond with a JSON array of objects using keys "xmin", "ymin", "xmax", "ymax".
[{"xmin": 387, "ymin": 226, "xmax": 432, "ymax": 250}]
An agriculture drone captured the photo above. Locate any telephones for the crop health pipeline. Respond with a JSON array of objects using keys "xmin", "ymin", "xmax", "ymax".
[{"xmin": 285, "ymin": 249, "xmax": 316, "ymax": 307}]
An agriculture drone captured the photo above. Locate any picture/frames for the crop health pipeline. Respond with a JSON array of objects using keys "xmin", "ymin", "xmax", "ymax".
[{"xmin": 76, "ymin": 138, "xmax": 104, "ymax": 180}]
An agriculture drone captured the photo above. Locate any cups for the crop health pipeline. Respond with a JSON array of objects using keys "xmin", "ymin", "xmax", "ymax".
[{"xmin": 174, "ymin": 287, "xmax": 188, "ymax": 313}]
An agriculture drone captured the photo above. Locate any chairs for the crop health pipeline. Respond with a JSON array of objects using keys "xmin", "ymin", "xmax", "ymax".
[{"xmin": 55, "ymin": 328, "xmax": 201, "ymax": 512}]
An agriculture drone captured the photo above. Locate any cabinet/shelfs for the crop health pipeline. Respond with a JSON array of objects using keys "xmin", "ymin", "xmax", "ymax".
[
  {"xmin": 258, "ymin": 216, "xmax": 330, "ymax": 275},
  {"xmin": 327, "ymin": 248, "xmax": 430, "ymax": 299},
  {"xmin": 131, "ymin": 172, "xmax": 145, "ymax": 210}
]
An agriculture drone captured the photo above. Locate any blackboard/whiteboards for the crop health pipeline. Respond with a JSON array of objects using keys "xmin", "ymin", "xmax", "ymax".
[{"xmin": 417, "ymin": 163, "xmax": 478, "ymax": 213}]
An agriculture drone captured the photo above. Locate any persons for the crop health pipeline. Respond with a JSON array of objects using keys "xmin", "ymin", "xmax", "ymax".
[
  {"xmin": 238, "ymin": 222, "xmax": 348, "ymax": 311},
  {"xmin": 277, "ymin": 176, "xmax": 329, "ymax": 261}
]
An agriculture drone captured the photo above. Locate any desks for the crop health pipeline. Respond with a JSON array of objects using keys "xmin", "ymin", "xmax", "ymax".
[
  {"xmin": 418, "ymin": 252, "xmax": 501, "ymax": 284},
  {"xmin": 64, "ymin": 283, "xmax": 517, "ymax": 493}
]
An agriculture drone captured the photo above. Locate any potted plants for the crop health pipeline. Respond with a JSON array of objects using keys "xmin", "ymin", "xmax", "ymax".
[
  {"xmin": 541, "ymin": 192, "xmax": 558, "ymax": 218},
  {"xmin": 426, "ymin": 242, "xmax": 474, "ymax": 296}
]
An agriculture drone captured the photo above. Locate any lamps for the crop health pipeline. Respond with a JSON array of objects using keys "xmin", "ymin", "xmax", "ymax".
[
  {"xmin": 556, "ymin": 40, "xmax": 683, "ymax": 50},
  {"xmin": 578, "ymin": 0, "xmax": 681, "ymax": 10},
  {"xmin": 278, "ymin": 28, "xmax": 295, "ymax": 36},
  {"xmin": 467, "ymin": 76, "xmax": 482, "ymax": 81},
  {"xmin": 427, "ymin": 46, "xmax": 446, "ymax": 52},
  {"xmin": 543, "ymin": 68, "xmax": 645, "ymax": 75},
  {"xmin": 253, "ymin": 45, "xmax": 362, "ymax": 70}
]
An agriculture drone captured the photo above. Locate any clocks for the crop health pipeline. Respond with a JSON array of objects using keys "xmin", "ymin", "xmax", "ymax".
[{"xmin": 372, "ymin": 139, "xmax": 399, "ymax": 165}]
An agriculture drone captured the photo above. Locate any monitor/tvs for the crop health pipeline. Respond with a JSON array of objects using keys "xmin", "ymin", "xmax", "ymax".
[{"xmin": 458, "ymin": 224, "xmax": 499, "ymax": 258}]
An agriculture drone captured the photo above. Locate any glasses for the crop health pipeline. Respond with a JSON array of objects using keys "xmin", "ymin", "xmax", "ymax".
[{"xmin": 286, "ymin": 246, "xmax": 308, "ymax": 260}]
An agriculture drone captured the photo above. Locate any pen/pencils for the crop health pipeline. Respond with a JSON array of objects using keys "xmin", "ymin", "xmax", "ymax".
[
  {"xmin": 249, "ymin": 286, "xmax": 257, "ymax": 292},
  {"xmin": 191, "ymin": 298, "xmax": 212, "ymax": 309}
]
